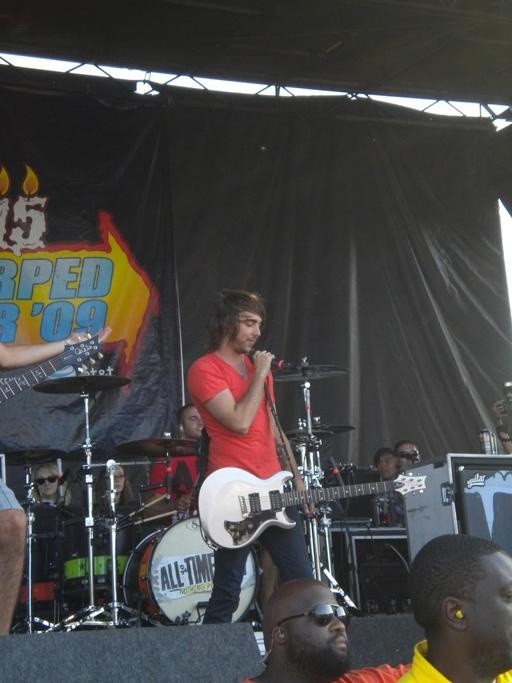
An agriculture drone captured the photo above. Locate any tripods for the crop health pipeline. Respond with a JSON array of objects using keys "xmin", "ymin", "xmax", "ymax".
[
  {"xmin": 10, "ymin": 393, "xmax": 165, "ymax": 633},
  {"xmin": 283, "ymin": 445, "xmax": 364, "ymax": 618}
]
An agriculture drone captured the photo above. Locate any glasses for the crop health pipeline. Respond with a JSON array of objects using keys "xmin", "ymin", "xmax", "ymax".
[
  {"xmin": 37, "ymin": 476, "xmax": 57, "ymax": 485},
  {"xmin": 277, "ymin": 602, "xmax": 350, "ymax": 627},
  {"xmin": 399, "ymin": 451, "xmax": 417, "ymax": 456}
]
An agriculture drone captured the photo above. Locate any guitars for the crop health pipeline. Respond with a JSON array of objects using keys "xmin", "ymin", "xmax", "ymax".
[
  {"xmin": 0, "ymin": 330, "xmax": 105, "ymax": 407},
  {"xmin": 192, "ymin": 460, "xmax": 427, "ymax": 551}
]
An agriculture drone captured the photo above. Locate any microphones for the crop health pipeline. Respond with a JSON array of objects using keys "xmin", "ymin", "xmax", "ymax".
[{"xmin": 247, "ymin": 349, "xmax": 293, "ymax": 369}]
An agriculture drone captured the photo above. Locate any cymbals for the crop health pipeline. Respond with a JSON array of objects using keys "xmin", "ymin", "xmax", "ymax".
[
  {"xmin": 284, "ymin": 425, "xmax": 357, "ymax": 440},
  {"xmin": 110, "ymin": 431, "xmax": 205, "ymax": 459},
  {"xmin": 271, "ymin": 364, "xmax": 352, "ymax": 383},
  {"xmin": 35, "ymin": 375, "xmax": 133, "ymax": 389}
]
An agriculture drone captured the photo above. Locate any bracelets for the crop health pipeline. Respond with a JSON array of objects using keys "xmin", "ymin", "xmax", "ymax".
[{"xmin": 291, "ymin": 473, "xmax": 305, "ymax": 483}]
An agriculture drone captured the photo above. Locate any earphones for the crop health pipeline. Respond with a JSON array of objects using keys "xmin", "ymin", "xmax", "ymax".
[
  {"xmin": 279, "ymin": 633, "xmax": 285, "ymax": 639},
  {"xmin": 455, "ymin": 610, "xmax": 464, "ymax": 619}
]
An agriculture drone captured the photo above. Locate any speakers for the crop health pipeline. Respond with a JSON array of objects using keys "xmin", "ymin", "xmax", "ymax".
[
  {"xmin": 349, "ymin": 518, "xmax": 408, "ymax": 615},
  {"xmin": 456, "ymin": 465, "xmax": 512, "ymax": 559}
]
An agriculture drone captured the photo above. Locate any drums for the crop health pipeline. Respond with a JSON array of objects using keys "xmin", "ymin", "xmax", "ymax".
[{"xmin": 122, "ymin": 517, "xmax": 257, "ymax": 628}]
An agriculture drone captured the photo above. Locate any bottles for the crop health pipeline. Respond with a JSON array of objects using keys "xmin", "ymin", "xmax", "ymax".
[{"xmin": 479, "ymin": 428, "xmax": 497, "ymax": 455}]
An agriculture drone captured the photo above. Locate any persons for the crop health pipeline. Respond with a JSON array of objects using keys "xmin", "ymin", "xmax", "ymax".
[
  {"xmin": 187, "ymin": 289, "xmax": 315, "ymax": 623},
  {"xmin": 238, "ymin": 577, "xmax": 415, "ymax": 683},
  {"xmin": 0, "ymin": 323, "xmax": 113, "ymax": 635},
  {"xmin": 146, "ymin": 401, "xmax": 206, "ymax": 525},
  {"xmin": 25, "ymin": 460, "xmax": 71, "ymax": 508},
  {"xmin": 377, "ymin": 438, "xmax": 421, "ymax": 614},
  {"xmin": 372, "ymin": 446, "xmax": 394, "ymax": 478},
  {"xmin": 396, "ymin": 534, "xmax": 512, "ymax": 682},
  {"xmin": 93, "ymin": 460, "xmax": 134, "ymax": 506}
]
